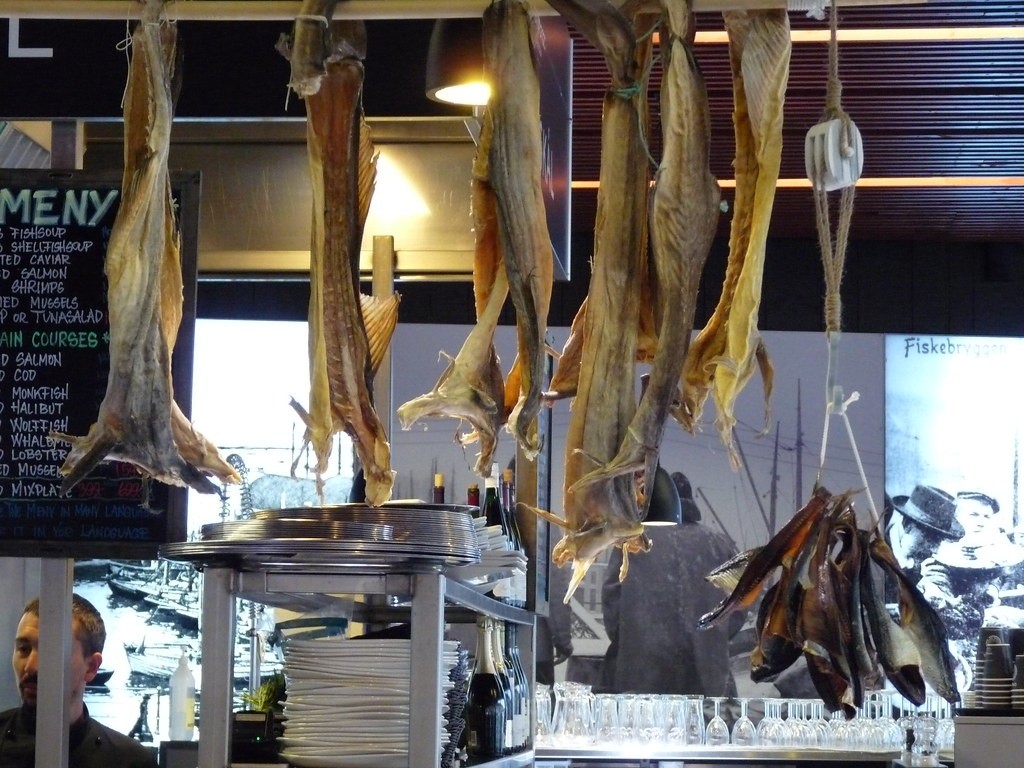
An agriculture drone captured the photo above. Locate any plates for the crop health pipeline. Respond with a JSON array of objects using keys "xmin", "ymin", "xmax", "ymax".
[
  {"xmin": 157, "ymin": 502, "xmax": 529, "ymax": 609},
  {"xmin": 276, "ymin": 639, "xmax": 462, "ymax": 768}
]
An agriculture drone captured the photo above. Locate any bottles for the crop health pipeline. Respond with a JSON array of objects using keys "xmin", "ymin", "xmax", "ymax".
[
  {"xmin": 465, "ymin": 616, "xmax": 532, "ymax": 766},
  {"xmin": 169, "ymin": 650, "xmax": 196, "ymax": 741},
  {"xmin": 432, "ymin": 473, "xmax": 446, "ymax": 503},
  {"xmin": 467, "ymin": 482, "xmax": 480, "ymax": 519},
  {"xmin": 482, "ymin": 461, "xmax": 520, "ymax": 553}
]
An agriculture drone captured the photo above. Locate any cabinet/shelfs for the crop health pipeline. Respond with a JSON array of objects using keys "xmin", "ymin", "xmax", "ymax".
[{"xmin": 197, "ymin": 567, "xmax": 537, "ymax": 768}]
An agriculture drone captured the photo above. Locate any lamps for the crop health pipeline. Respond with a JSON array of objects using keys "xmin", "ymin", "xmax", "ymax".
[{"xmin": 425, "ymin": 19, "xmax": 491, "ymax": 106}]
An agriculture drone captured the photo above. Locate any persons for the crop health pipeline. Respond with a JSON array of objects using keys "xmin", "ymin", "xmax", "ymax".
[
  {"xmin": 592, "ymin": 474, "xmax": 749, "ymax": 693},
  {"xmin": 442, "ymin": 454, "xmax": 574, "ymax": 723},
  {"xmin": 0, "ymin": 592, "xmax": 156, "ymax": 768},
  {"xmin": 887, "ymin": 486, "xmax": 1023, "ymax": 629}
]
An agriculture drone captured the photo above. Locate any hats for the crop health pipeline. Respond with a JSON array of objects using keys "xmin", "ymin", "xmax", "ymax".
[
  {"xmin": 891, "ymin": 485, "xmax": 965, "ymax": 540},
  {"xmin": 958, "ymin": 491, "xmax": 1000, "ymax": 513},
  {"xmin": 671, "ymin": 472, "xmax": 702, "ymax": 522},
  {"xmin": 934, "ymin": 520, "xmax": 1024, "ymax": 571}
]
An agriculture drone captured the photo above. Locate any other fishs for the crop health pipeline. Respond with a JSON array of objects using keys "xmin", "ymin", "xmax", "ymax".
[
  {"xmin": 46, "ymin": 0, "xmax": 792, "ymax": 604},
  {"xmin": 694, "ymin": 486, "xmax": 961, "ymax": 713}
]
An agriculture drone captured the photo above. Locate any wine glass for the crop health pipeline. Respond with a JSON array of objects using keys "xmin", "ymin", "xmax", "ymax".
[{"xmin": 705, "ymin": 689, "xmax": 956, "ymax": 768}]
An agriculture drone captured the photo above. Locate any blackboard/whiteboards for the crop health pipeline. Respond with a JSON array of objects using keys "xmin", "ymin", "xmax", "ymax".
[{"xmin": 1, "ymin": 167, "xmax": 202, "ymax": 558}]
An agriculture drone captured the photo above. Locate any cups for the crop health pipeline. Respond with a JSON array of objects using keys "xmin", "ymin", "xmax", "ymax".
[
  {"xmin": 974, "ymin": 627, "xmax": 1024, "ymax": 710},
  {"xmin": 593, "ymin": 693, "xmax": 704, "ymax": 749}
]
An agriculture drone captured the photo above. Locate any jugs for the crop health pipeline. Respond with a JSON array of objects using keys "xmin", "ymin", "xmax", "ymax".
[{"xmin": 535, "ymin": 681, "xmax": 594, "ymax": 748}]
